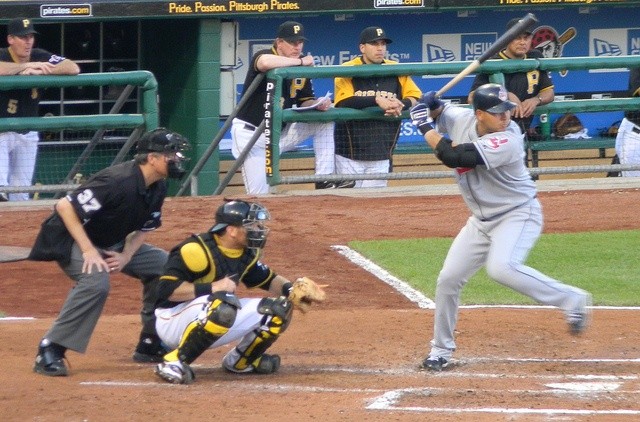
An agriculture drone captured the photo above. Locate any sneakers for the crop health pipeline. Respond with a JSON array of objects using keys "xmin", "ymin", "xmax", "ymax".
[
  {"xmin": 423, "ymin": 346, "xmax": 452, "ymax": 371},
  {"xmin": 132, "ymin": 332, "xmax": 167, "ymax": 363},
  {"xmin": 154, "ymin": 360, "xmax": 194, "ymax": 384},
  {"xmin": 567, "ymin": 312, "xmax": 584, "ymax": 329},
  {"xmin": 32, "ymin": 338, "xmax": 72, "ymax": 376},
  {"xmin": 315, "ymin": 180, "xmax": 356, "ymax": 187},
  {"xmin": 256, "ymin": 353, "xmax": 279, "ymax": 375}
]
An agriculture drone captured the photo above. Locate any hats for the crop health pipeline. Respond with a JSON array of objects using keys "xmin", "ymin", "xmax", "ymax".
[
  {"xmin": 360, "ymin": 26, "xmax": 392, "ymax": 44},
  {"xmin": 507, "ymin": 17, "xmax": 532, "ymax": 33},
  {"xmin": 8, "ymin": 17, "xmax": 38, "ymax": 36},
  {"xmin": 278, "ymin": 21, "xmax": 309, "ymax": 42}
]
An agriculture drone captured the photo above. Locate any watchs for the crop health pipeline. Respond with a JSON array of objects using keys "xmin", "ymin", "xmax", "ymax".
[{"xmin": 536, "ymin": 95, "xmax": 542, "ymax": 104}]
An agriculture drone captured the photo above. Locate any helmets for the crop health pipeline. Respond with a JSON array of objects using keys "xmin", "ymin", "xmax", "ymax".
[
  {"xmin": 136, "ymin": 128, "xmax": 192, "ymax": 180},
  {"xmin": 208, "ymin": 200, "xmax": 271, "ymax": 249},
  {"xmin": 472, "ymin": 83, "xmax": 518, "ymax": 114}
]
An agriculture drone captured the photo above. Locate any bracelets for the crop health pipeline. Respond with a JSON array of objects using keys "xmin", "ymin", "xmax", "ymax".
[
  {"xmin": 398, "ymin": 98, "xmax": 412, "ymax": 111},
  {"xmin": 299, "ymin": 58, "xmax": 304, "ymax": 66},
  {"xmin": 195, "ymin": 282, "xmax": 213, "ymax": 300},
  {"xmin": 282, "ymin": 282, "xmax": 294, "ymax": 298}
]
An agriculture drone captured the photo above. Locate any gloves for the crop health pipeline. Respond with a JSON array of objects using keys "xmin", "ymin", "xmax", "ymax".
[
  {"xmin": 419, "ymin": 91, "xmax": 444, "ymax": 110},
  {"xmin": 409, "ymin": 102, "xmax": 434, "ymax": 134}
]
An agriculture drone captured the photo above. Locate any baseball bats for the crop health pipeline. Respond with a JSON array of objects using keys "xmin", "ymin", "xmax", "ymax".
[{"xmin": 434, "ymin": 12, "xmax": 538, "ymax": 98}]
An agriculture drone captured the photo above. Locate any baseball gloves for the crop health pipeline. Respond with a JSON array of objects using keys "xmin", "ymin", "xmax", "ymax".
[
  {"xmin": 286, "ymin": 276, "xmax": 329, "ymax": 314},
  {"xmin": 553, "ymin": 112, "xmax": 583, "ymax": 139}
]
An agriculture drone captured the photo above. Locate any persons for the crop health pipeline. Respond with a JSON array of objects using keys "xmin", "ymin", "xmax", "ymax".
[
  {"xmin": 0, "ymin": 16, "xmax": 83, "ymax": 203},
  {"xmin": 331, "ymin": 24, "xmax": 426, "ymax": 189},
  {"xmin": 615, "ymin": 85, "xmax": 638, "ymax": 177},
  {"xmin": 151, "ymin": 197, "xmax": 326, "ymax": 386},
  {"xmin": 409, "ymin": 81, "xmax": 594, "ymax": 375},
  {"xmin": 529, "ymin": 25, "xmax": 562, "ymax": 63},
  {"xmin": 30, "ymin": 125, "xmax": 194, "ymax": 379},
  {"xmin": 466, "ymin": 15, "xmax": 557, "ymax": 181},
  {"xmin": 229, "ymin": 20, "xmax": 357, "ymax": 197}
]
